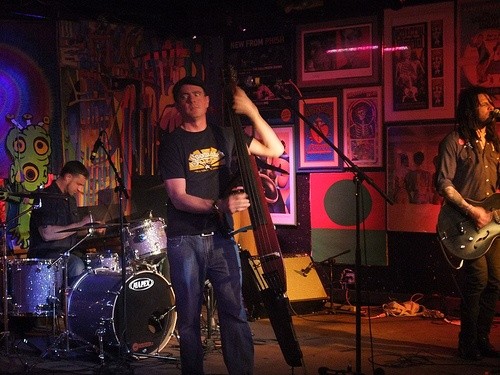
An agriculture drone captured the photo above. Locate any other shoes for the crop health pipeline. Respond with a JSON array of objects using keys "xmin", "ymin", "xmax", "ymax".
[
  {"xmin": 478, "ymin": 336, "xmax": 500, "ymax": 358},
  {"xmin": 460, "ymin": 333, "xmax": 481, "ymax": 360}
]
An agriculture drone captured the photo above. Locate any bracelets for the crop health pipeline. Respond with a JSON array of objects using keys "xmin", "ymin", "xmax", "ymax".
[{"xmin": 465, "ymin": 205, "xmax": 472, "ymax": 215}]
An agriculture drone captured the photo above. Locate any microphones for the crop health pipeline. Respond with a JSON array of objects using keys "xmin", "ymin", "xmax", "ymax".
[
  {"xmin": 304, "ymin": 263, "xmax": 313, "ymax": 274},
  {"xmin": 89, "ymin": 132, "xmax": 103, "ymax": 161},
  {"xmin": 493, "ymin": 108, "xmax": 500, "ymax": 117},
  {"xmin": 246, "ymin": 76, "xmax": 291, "ymax": 88}
]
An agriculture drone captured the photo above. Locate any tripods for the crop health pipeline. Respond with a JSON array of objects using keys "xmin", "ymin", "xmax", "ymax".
[{"xmin": 0, "ymin": 143, "xmax": 181, "ymax": 372}]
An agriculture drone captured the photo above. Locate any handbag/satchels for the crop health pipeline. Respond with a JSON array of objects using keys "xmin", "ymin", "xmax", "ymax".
[{"xmin": 382, "ymin": 301, "xmax": 420, "ymax": 317}]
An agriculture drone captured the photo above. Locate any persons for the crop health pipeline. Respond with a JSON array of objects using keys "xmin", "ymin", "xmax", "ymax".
[
  {"xmin": 157, "ymin": 76, "xmax": 285, "ymax": 375},
  {"xmin": 432, "ymin": 86, "xmax": 500, "ymax": 361},
  {"xmin": 26, "ymin": 160, "xmax": 106, "ymax": 285}
]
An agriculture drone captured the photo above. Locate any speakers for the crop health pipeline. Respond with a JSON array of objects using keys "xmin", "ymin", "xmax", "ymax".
[{"xmin": 242, "ymin": 253, "xmax": 328, "ymax": 319}]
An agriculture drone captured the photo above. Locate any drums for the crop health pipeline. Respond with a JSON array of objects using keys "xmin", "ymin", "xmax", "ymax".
[{"xmin": 1, "ymin": 216, "xmax": 177, "ymax": 362}]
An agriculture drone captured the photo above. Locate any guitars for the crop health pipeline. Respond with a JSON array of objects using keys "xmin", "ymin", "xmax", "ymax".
[{"xmin": 437, "ymin": 192, "xmax": 500, "ymax": 264}]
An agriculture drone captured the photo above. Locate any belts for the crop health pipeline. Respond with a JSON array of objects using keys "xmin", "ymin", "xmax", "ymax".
[{"xmin": 201, "ymin": 231, "xmax": 217, "ymax": 237}]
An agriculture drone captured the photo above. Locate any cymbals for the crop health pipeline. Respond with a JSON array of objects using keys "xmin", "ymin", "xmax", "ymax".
[
  {"xmin": 54, "ymin": 222, "xmax": 130, "ymax": 234},
  {"xmin": 6, "ymin": 189, "xmax": 72, "ymax": 199}
]
